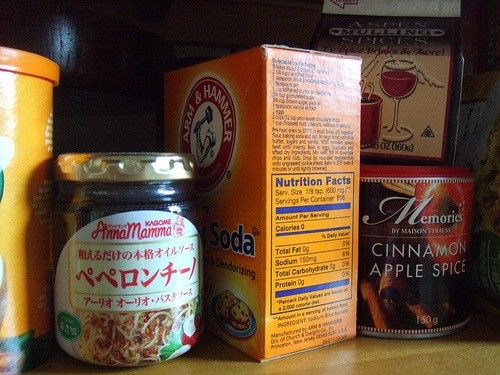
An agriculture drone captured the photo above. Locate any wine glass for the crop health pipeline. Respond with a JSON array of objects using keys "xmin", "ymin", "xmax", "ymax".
[{"xmin": 381, "ymin": 57, "xmax": 419, "ymax": 142}]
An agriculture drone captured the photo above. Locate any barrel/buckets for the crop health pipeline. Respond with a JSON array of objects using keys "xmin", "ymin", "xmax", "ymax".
[{"xmin": 360, "ymin": 91, "xmax": 383, "ymax": 149}]
